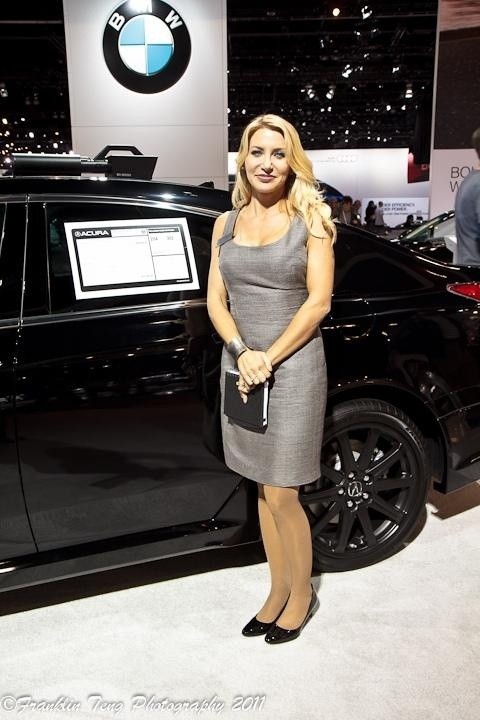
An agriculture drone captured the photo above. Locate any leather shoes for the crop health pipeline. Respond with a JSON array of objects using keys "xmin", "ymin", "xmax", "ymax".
[{"xmin": 241, "ymin": 582, "xmax": 321, "ymax": 645}]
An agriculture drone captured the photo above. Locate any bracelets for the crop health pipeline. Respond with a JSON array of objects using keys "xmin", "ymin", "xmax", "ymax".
[{"xmin": 225, "ymin": 337, "xmax": 254, "ymax": 361}]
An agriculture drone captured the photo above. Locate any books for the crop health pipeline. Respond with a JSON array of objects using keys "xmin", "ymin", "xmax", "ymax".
[{"xmin": 221, "ymin": 367, "xmax": 269, "ymax": 430}]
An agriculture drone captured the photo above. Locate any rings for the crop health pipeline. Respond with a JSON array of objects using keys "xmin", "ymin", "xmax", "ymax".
[
  {"xmin": 250, "ymin": 375, "xmax": 258, "ymax": 383},
  {"xmin": 263, "ymin": 370, "xmax": 269, "ymax": 377},
  {"xmin": 242, "ymin": 382, "xmax": 246, "ymax": 389}
]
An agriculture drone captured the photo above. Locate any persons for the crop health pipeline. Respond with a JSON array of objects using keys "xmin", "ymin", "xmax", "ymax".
[
  {"xmin": 449, "ymin": 126, "xmax": 479, "ymax": 269},
  {"xmin": 325, "ymin": 194, "xmax": 415, "ymax": 232},
  {"xmin": 202, "ymin": 112, "xmax": 342, "ymax": 646}
]
